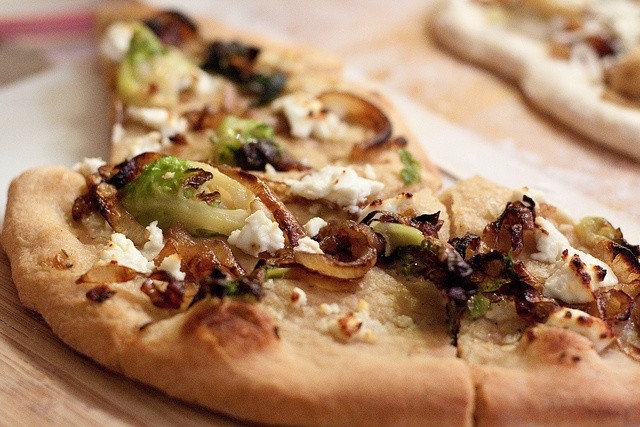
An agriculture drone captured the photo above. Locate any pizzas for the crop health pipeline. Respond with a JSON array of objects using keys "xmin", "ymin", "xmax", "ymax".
[
  {"xmin": 428, "ymin": 0, "xmax": 640, "ymax": 163},
  {"xmin": 0, "ymin": 0, "xmax": 640, "ymax": 427}
]
